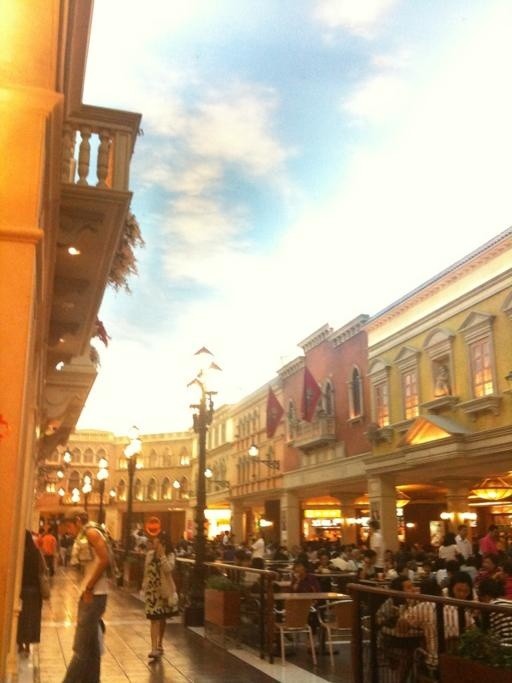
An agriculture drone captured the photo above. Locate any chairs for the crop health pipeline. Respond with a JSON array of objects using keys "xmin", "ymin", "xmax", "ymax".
[{"xmin": 123, "ymin": 533, "xmax": 486, "ymax": 682}]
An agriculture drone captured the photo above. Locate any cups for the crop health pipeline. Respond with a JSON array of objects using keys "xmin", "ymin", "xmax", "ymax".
[{"xmin": 377, "ymin": 572, "xmax": 384, "ymax": 581}]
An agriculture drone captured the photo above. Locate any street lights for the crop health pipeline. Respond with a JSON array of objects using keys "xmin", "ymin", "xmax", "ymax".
[
  {"xmin": 72, "ymin": 487, "xmax": 80, "ymax": 506},
  {"xmin": 97, "ymin": 456, "xmax": 109, "ymax": 530},
  {"xmin": 118, "ymin": 425, "xmax": 142, "ymax": 586},
  {"xmin": 184, "ymin": 345, "xmax": 222, "ymax": 627},
  {"xmin": 82, "ymin": 476, "xmax": 92, "ymax": 512}
]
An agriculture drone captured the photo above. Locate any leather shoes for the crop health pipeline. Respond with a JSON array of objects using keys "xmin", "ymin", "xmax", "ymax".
[
  {"xmin": 156, "ymin": 644, "xmax": 163, "ymax": 656},
  {"xmin": 148, "ymin": 649, "xmax": 161, "ymax": 659}
]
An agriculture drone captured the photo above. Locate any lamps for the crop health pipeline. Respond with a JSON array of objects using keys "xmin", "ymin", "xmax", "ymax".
[
  {"xmin": 33, "ymin": 448, "xmax": 73, "ymax": 497},
  {"xmin": 202, "ymin": 468, "xmax": 230, "ymax": 488},
  {"xmin": 248, "ymin": 445, "xmax": 280, "ymax": 470}
]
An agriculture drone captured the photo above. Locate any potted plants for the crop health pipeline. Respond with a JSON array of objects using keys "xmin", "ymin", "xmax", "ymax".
[{"xmin": 438, "ymin": 624, "xmax": 511, "ymax": 683}]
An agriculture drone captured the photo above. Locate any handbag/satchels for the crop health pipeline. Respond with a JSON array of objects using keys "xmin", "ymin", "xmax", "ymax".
[{"xmin": 40, "ymin": 566, "xmax": 52, "ymax": 600}]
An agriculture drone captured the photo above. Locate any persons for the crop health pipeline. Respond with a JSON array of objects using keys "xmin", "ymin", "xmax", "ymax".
[
  {"xmin": 141, "ymin": 529, "xmax": 179, "ymax": 660},
  {"xmin": 276, "ymin": 557, "xmax": 322, "ymax": 641},
  {"xmin": 266, "ymin": 537, "xmax": 378, "ymax": 592},
  {"xmin": 382, "ymin": 524, "xmax": 511, "ymax": 600},
  {"xmin": 367, "ymin": 520, "xmax": 385, "ymax": 572},
  {"xmin": 207, "ymin": 557, "xmax": 280, "ymax": 610},
  {"xmin": 123, "ymin": 529, "xmax": 265, "ymax": 581},
  {"xmin": 36, "ymin": 526, "xmax": 77, "ymax": 576},
  {"xmin": 17, "ymin": 528, "xmax": 44, "ymax": 653},
  {"xmin": 375, "ymin": 572, "xmax": 511, "ymax": 682},
  {"xmin": 63, "ymin": 506, "xmax": 121, "ymax": 683}
]
